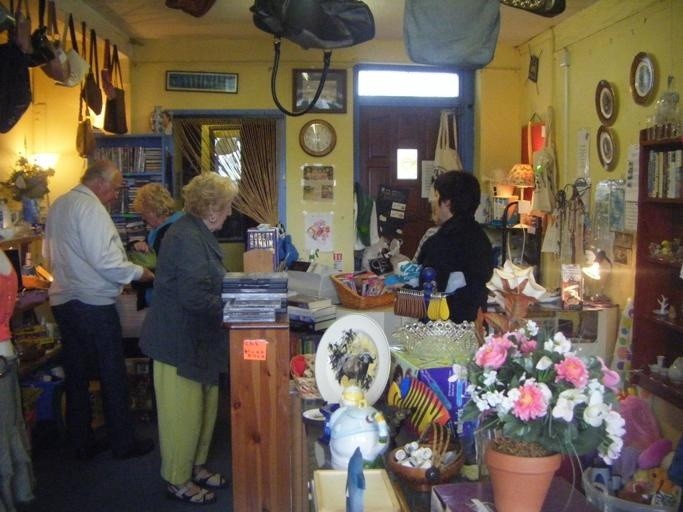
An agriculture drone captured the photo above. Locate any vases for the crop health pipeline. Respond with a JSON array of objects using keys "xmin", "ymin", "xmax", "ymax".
[
  {"xmin": 22, "ymin": 198, "xmax": 40, "ymax": 225},
  {"xmin": 481, "ymin": 441, "xmax": 563, "ymax": 511}
]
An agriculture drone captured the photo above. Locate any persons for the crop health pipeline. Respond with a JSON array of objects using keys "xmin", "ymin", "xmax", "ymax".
[
  {"xmin": 126, "ymin": 182, "xmax": 184, "ymax": 313},
  {"xmin": 0, "ymin": 249, "xmax": 39, "ymax": 511},
  {"xmin": 40, "ymin": 160, "xmax": 156, "ymax": 461},
  {"xmin": 162, "ymin": 112, "xmax": 172, "ymax": 134},
  {"xmin": 138, "ymin": 169, "xmax": 238, "ymax": 503},
  {"xmin": 392, "ymin": 169, "xmax": 495, "ymax": 337}
]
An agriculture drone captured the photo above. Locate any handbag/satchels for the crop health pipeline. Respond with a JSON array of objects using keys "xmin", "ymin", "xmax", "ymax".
[
  {"xmin": 80, "ymin": 119, "xmax": 105, "ymax": 156},
  {"xmin": 249, "ymin": 0, "xmax": 375, "ymax": 49},
  {"xmin": 428, "ymin": 146, "xmax": 462, "ymax": 201},
  {"xmin": 404, "ymin": 0, "xmax": 500, "ymax": 70},
  {"xmin": 0, "ymin": 1, "xmax": 128, "ymax": 135},
  {"xmin": 529, "ymin": 149, "xmax": 558, "ymax": 212}
]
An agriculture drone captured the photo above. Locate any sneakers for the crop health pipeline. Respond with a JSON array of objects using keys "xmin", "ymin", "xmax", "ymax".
[{"xmin": 126, "ymin": 438, "xmax": 154, "ymax": 460}]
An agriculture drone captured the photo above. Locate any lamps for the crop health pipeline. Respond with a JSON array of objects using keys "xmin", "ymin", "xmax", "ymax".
[
  {"xmin": 581, "ymin": 250, "xmax": 617, "ymax": 306},
  {"xmin": 506, "ymin": 164, "xmax": 536, "ymax": 228}
]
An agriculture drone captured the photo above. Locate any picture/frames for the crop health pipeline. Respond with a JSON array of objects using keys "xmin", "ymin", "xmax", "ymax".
[
  {"xmin": 290, "ymin": 68, "xmax": 347, "ymax": 114},
  {"xmin": 298, "ymin": 119, "xmax": 337, "ymax": 158},
  {"xmin": 164, "ymin": 71, "xmax": 238, "ymax": 95}
]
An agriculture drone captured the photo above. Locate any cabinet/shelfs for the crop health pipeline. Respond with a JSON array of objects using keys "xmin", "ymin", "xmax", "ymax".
[
  {"xmin": 87, "ymin": 135, "xmax": 177, "ymax": 339},
  {"xmin": 630, "ymin": 127, "xmax": 682, "ymax": 451}
]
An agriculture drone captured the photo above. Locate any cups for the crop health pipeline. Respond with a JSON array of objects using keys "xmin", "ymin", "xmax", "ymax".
[{"xmin": 643, "ymin": 102, "xmax": 683, "ymax": 141}]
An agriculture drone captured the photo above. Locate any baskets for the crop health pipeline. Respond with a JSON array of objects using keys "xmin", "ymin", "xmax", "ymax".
[
  {"xmin": 329, "ymin": 272, "xmax": 396, "ymax": 309},
  {"xmin": 386, "ymin": 424, "xmax": 465, "ymax": 484}
]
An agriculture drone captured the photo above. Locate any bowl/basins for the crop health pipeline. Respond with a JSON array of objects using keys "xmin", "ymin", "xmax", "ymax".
[{"xmin": 0, "ymin": 227, "xmax": 19, "ymax": 239}]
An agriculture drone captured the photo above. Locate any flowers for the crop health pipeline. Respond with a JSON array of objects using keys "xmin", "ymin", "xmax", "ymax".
[
  {"xmin": 447, "ymin": 319, "xmax": 624, "ymax": 467},
  {"xmin": 0, "ymin": 156, "xmax": 55, "ymax": 202}
]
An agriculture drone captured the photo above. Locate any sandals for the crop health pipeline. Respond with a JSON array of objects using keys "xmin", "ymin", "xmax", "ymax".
[
  {"xmin": 168, "ymin": 480, "xmax": 216, "ymax": 504},
  {"xmin": 191, "ymin": 469, "xmax": 227, "ymax": 487}
]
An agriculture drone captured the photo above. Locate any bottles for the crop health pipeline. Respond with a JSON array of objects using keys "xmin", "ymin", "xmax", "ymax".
[
  {"xmin": 474, "ymin": 192, "xmax": 492, "ymax": 225},
  {"xmin": 150, "ymin": 105, "xmax": 167, "ymax": 135},
  {"xmin": 648, "ymin": 354, "xmax": 683, "ymax": 383}
]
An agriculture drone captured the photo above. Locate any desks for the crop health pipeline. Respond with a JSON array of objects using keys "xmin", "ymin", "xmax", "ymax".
[
  {"xmin": 478, "ymin": 222, "xmax": 540, "ymax": 268},
  {"xmin": 429, "ymin": 473, "xmax": 600, "ymax": 511}
]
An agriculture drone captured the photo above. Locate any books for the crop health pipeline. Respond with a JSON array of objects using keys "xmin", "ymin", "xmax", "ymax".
[
  {"xmin": 116, "ymin": 289, "xmax": 149, "ymax": 337},
  {"xmin": 220, "ymin": 223, "xmax": 337, "ymax": 356},
  {"xmin": 93, "ymin": 146, "xmax": 161, "ymax": 250},
  {"xmin": 647, "ymin": 149, "xmax": 682, "ymax": 199}
]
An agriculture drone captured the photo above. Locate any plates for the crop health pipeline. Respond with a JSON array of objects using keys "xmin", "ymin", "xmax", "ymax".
[
  {"xmin": 629, "ymin": 51, "xmax": 657, "ymax": 106},
  {"xmin": 300, "ymin": 408, "xmax": 327, "ymax": 422},
  {"xmin": 596, "ymin": 125, "xmax": 617, "ymax": 173},
  {"xmin": 313, "ymin": 313, "xmax": 390, "ymax": 408},
  {"xmin": 594, "ymin": 80, "xmax": 617, "ymax": 126}
]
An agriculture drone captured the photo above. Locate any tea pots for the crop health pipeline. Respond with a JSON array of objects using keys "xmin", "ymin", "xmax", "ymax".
[{"xmin": 328, "ymin": 385, "xmax": 390, "ymax": 470}]
